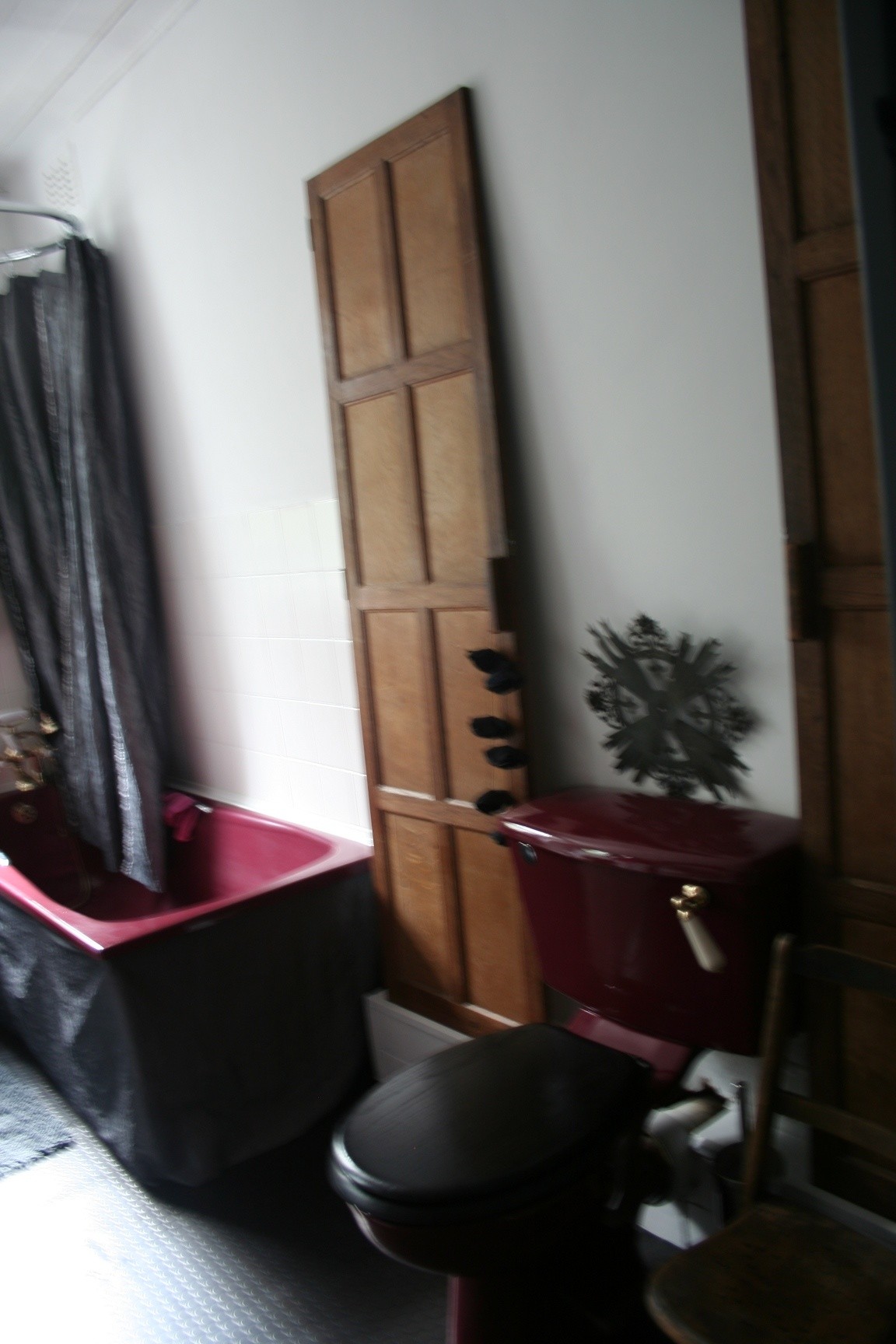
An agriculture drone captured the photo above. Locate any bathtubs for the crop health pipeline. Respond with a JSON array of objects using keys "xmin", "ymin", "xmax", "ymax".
[{"xmin": 0, "ymin": 781, "xmax": 375, "ymax": 959}]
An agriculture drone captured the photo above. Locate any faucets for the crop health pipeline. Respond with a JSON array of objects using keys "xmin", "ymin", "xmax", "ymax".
[{"xmin": 0, "ymin": 709, "xmax": 59, "ymax": 793}]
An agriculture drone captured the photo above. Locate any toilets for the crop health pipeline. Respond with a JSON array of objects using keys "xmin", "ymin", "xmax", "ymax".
[{"xmin": 324, "ymin": 783, "xmax": 807, "ymax": 1344}]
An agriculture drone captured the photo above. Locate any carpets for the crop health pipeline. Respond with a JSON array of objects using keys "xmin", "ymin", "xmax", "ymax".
[{"xmin": 0, "ymin": 1060, "xmax": 74, "ymax": 1176}]
